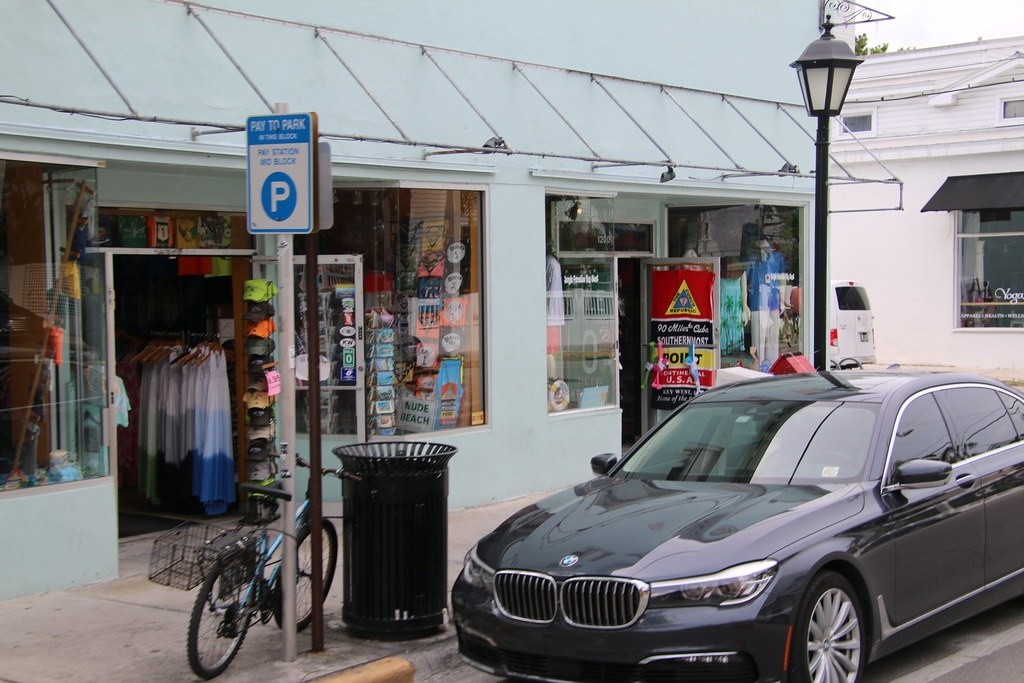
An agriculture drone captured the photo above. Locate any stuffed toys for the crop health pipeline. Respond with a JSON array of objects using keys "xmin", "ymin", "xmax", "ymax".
[
  {"xmin": 684, "ymin": 341, "xmax": 700, "ymax": 395},
  {"xmin": 641, "ymin": 341, "xmax": 669, "ymax": 389}
]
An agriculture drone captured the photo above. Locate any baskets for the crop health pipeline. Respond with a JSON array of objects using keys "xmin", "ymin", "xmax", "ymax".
[{"xmin": 147, "ymin": 520, "xmax": 266, "ymax": 604}]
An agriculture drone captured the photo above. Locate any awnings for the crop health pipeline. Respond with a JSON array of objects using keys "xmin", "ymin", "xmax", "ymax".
[{"xmin": 921, "ymin": 172, "xmax": 1024, "ymax": 212}]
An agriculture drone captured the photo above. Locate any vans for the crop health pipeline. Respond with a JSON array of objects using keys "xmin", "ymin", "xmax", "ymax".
[{"xmin": 828, "ymin": 276, "xmax": 875, "ymax": 367}]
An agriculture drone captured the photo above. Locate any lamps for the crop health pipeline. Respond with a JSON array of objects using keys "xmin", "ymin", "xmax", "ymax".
[
  {"xmin": 779, "ymin": 163, "xmax": 800, "ymax": 177},
  {"xmin": 660, "ymin": 164, "xmax": 676, "ymax": 183},
  {"xmin": 482, "ymin": 137, "xmax": 511, "ymax": 156},
  {"xmin": 565, "ymin": 199, "xmax": 581, "ymax": 221}
]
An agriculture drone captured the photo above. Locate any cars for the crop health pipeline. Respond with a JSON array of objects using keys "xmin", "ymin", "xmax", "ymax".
[
  {"xmin": 0, "ymin": 344, "xmax": 153, "ymax": 484},
  {"xmin": 451, "ymin": 358, "xmax": 1024, "ymax": 683}
]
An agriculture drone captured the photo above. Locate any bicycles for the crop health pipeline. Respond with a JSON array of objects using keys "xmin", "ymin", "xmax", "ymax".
[{"xmin": 147, "ymin": 442, "xmax": 363, "ymax": 679}]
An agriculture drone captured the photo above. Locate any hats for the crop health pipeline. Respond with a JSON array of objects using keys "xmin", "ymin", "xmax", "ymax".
[
  {"xmin": 243, "ymin": 301, "xmax": 275, "ymax": 319},
  {"xmin": 243, "ymin": 354, "xmax": 280, "ymax": 523},
  {"xmin": 243, "ymin": 279, "xmax": 278, "ymax": 302},
  {"xmin": 247, "ymin": 319, "xmax": 276, "ymax": 338},
  {"xmin": 246, "ymin": 336, "xmax": 275, "ymax": 355}
]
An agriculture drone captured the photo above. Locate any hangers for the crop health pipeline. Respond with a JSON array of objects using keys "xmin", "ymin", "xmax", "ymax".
[{"xmin": 118, "ymin": 330, "xmax": 237, "ymax": 368}]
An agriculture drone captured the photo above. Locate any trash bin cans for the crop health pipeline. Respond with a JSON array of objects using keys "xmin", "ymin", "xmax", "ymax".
[{"xmin": 331, "ymin": 441, "xmax": 458, "ymax": 642}]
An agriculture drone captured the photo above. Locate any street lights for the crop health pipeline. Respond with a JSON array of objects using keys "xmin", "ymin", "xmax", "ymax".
[{"xmin": 789, "ymin": 14, "xmax": 864, "ymax": 369}]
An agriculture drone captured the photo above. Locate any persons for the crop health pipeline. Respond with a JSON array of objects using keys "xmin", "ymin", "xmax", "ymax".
[{"xmin": 742, "ymin": 237, "xmax": 787, "ymax": 365}]
